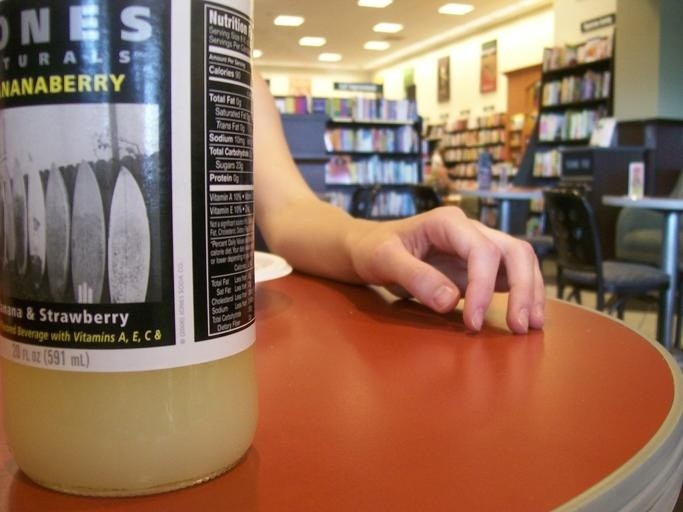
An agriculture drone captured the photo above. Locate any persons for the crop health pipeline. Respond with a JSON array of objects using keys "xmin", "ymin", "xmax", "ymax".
[{"xmin": 250, "ymin": 64, "xmax": 546, "ymax": 335}]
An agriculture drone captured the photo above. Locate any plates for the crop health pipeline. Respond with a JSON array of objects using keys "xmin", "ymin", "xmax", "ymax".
[
  {"xmin": 530, "ymin": 57, "xmax": 616, "ymax": 182},
  {"xmin": 423, "ymin": 124, "xmax": 504, "ymax": 182},
  {"xmin": 324, "ymin": 116, "xmax": 421, "ymax": 219}
]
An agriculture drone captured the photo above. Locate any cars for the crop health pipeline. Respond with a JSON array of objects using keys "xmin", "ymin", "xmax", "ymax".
[
  {"xmin": 627, "ymin": 161, "xmax": 644, "ymax": 200},
  {"xmin": 476, "ymin": 152, "xmax": 492, "ymax": 190}
]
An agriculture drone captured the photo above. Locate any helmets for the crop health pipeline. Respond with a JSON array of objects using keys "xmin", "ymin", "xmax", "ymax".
[{"xmin": 253, "ymin": 247, "xmax": 293, "ymax": 285}]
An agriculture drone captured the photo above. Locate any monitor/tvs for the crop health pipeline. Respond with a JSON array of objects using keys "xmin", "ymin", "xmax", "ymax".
[
  {"xmin": 272, "ymin": 92, "xmax": 421, "ymax": 218},
  {"xmin": 508, "ymin": 36, "xmax": 616, "ymax": 178},
  {"xmin": 415, "ymin": 102, "xmax": 507, "ymax": 192}
]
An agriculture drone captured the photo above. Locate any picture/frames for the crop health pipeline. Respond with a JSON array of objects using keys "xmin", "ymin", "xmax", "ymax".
[
  {"xmin": 462, "ymin": 187, "xmax": 539, "ymax": 241},
  {"xmin": 601, "ymin": 191, "xmax": 681, "ymax": 350},
  {"xmin": 0, "ymin": 255, "xmax": 681, "ymax": 511}
]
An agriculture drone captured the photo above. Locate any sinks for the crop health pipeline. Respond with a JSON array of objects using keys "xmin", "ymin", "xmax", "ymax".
[{"xmin": 615, "ymin": 167, "xmax": 681, "ymax": 322}]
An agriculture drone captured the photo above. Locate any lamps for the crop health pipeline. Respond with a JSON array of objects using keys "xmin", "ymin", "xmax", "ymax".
[
  {"xmin": 348, "ymin": 184, "xmax": 382, "ymax": 219},
  {"xmin": 545, "ymin": 187, "xmax": 668, "ymax": 346},
  {"xmin": 406, "ymin": 183, "xmax": 443, "ymax": 215}
]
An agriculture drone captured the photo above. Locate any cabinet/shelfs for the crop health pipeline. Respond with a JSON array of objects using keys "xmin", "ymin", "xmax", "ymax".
[{"xmin": 0, "ymin": 0, "xmax": 261, "ymax": 501}]
